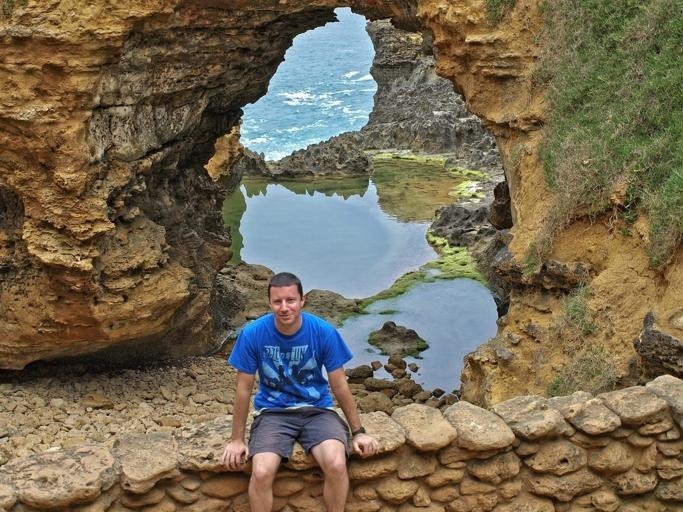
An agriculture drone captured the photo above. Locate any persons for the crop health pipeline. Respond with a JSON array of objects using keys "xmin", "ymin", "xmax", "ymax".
[{"xmin": 221, "ymin": 272, "xmax": 380, "ymax": 512}]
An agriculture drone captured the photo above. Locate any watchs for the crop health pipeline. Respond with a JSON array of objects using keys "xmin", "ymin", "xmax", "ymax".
[{"xmin": 352, "ymin": 426, "xmax": 365, "ymax": 437}]
